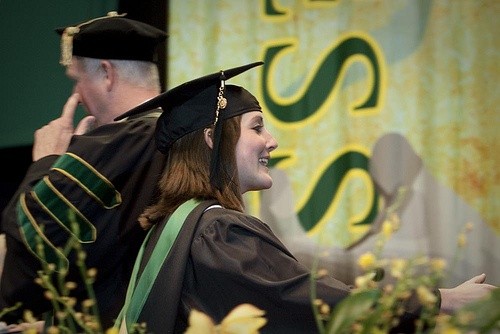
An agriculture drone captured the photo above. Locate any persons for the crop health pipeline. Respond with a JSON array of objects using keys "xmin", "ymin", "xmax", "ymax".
[
  {"xmin": 0, "ymin": 10, "xmax": 170, "ymax": 333},
  {"xmin": 111, "ymin": 60, "xmax": 500, "ymax": 334}
]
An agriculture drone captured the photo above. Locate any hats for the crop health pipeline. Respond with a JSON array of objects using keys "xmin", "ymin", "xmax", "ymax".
[
  {"xmin": 59, "ymin": 12, "xmax": 170, "ymax": 67},
  {"xmin": 113, "ymin": 59, "xmax": 267, "ymax": 189}
]
